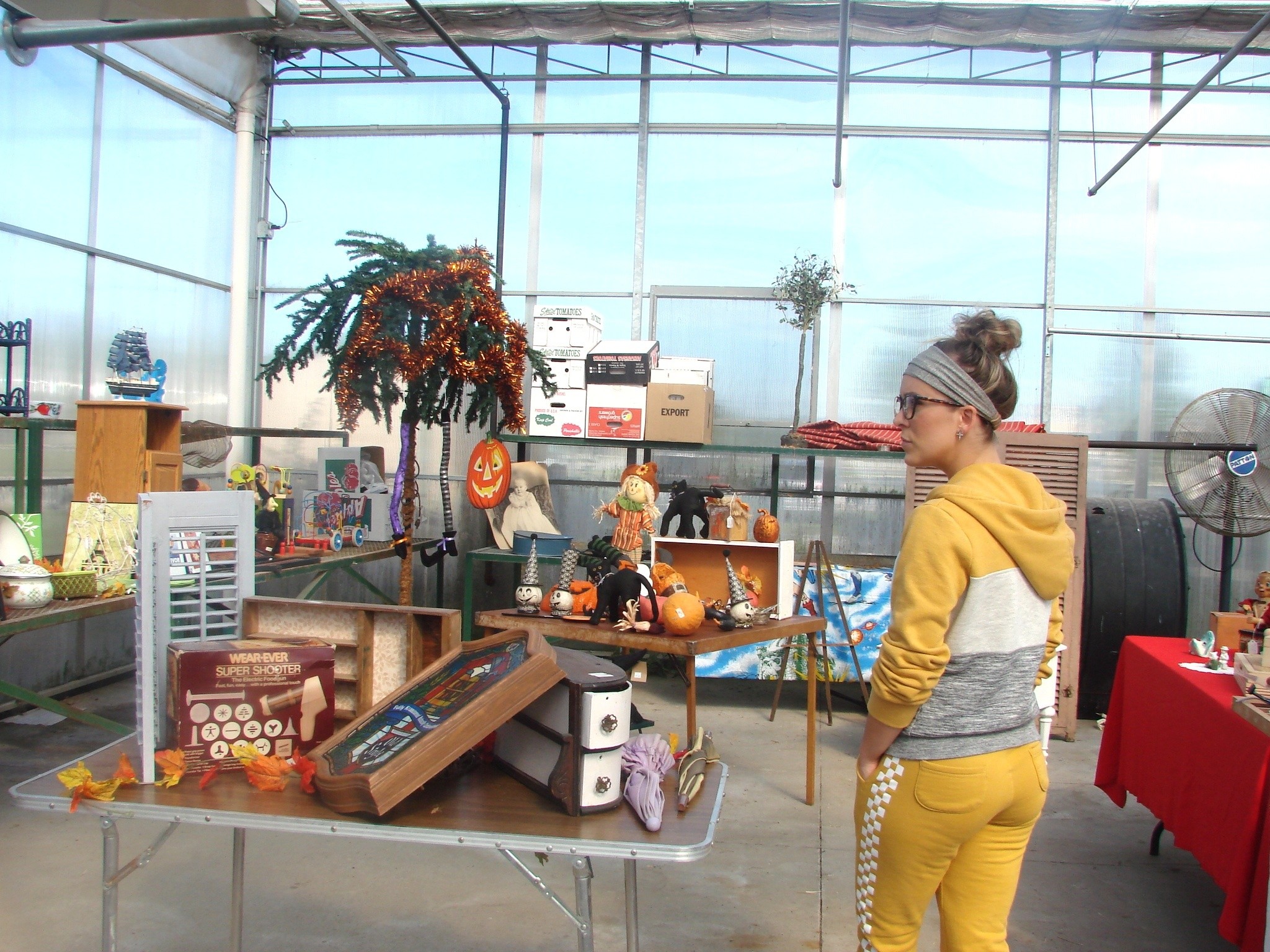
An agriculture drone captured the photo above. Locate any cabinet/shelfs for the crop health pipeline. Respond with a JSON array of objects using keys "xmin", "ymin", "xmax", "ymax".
[
  {"xmin": 493, "ymin": 646, "xmax": 634, "ymax": 817},
  {"xmin": 72, "ymin": 400, "xmax": 189, "ymax": 503},
  {"xmin": 651, "ymin": 537, "xmax": 795, "ymax": 620}
]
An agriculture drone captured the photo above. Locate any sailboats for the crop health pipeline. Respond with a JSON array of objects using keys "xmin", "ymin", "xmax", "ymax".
[{"xmin": 104, "ymin": 325, "xmax": 160, "ymax": 403}]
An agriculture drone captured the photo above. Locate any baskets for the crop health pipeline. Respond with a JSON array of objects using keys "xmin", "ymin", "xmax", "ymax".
[{"xmin": 51, "ymin": 568, "xmax": 98, "ymax": 601}]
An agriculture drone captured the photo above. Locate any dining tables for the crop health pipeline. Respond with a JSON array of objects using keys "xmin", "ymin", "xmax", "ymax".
[{"xmin": 474, "ymin": 608, "xmax": 828, "ymax": 806}]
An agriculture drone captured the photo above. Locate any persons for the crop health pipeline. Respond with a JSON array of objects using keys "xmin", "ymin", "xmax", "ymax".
[
  {"xmin": 1234, "ymin": 570, "xmax": 1270, "ymax": 652},
  {"xmin": 498, "ymin": 478, "xmax": 557, "ymax": 546},
  {"xmin": 591, "ymin": 461, "xmax": 661, "ymax": 561},
  {"xmin": 852, "ymin": 309, "xmax": 1073, "ymax": 951}
]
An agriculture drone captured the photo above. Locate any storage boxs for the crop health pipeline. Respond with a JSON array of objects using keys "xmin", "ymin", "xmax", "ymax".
[
  {"xmin": 527, "ymin": 305, "xmax": 717, "ymax": 445},
  {"xmin": 242, "ymin": 595, "xmax": 462, "ymax": 736},
  {"xmin": 167, "ymin": 636, "xmax": 337, "ymax": 777},
  {"xmin": 301, "ymin": 446, "xmax": 404, "ymax": 542}
]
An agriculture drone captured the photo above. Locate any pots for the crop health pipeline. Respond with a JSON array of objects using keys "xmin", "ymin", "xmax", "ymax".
[{"xmin": 0, "ymin": 556, "xmax": 54, "ymax": 609}]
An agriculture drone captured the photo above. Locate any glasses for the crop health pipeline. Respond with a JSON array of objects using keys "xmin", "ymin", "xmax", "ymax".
[{"xmin": 893, "ymin": 395, "xmax": 963, "ymax": 420}]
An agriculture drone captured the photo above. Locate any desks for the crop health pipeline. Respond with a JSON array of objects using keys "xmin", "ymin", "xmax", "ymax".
[
  {"xmin": 1122, "ymin": 635, "xmax": 1270, "ymax": 952},
  {"xmin": 462, "ymin": 545, "xmax": 652, "ymax": 659},
  {"xmin": 0, "ymin": 536, "xmax": 445, "ymax": 740},
  {"xmin": 8, "ymin": 732, "xmax": 729, "ymax": 952}
]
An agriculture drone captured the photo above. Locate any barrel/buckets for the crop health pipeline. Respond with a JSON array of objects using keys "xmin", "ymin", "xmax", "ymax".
[{"xmin": 256, "ymin": 532, "xmax": 278, "ymax": 555}]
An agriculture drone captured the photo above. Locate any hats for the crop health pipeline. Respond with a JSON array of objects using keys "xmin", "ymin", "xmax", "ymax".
[{"xmin": 620, "ymin": 461, "xmax": 660, "ymax": 502}]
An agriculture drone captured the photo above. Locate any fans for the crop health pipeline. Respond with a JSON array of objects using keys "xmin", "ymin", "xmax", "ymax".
[{"xmin": 1163, "ymin": 388, "xmax": 1270, "ymax": 613}]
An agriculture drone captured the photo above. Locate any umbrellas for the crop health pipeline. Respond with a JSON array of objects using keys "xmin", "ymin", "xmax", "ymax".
[
  {"xmin": 673, "ymin": 726, "xmax": 720, "ymax": 812},
  {"xmin": 620, "ymin": 732, "xmax": 677, "ymax": 831}
]
